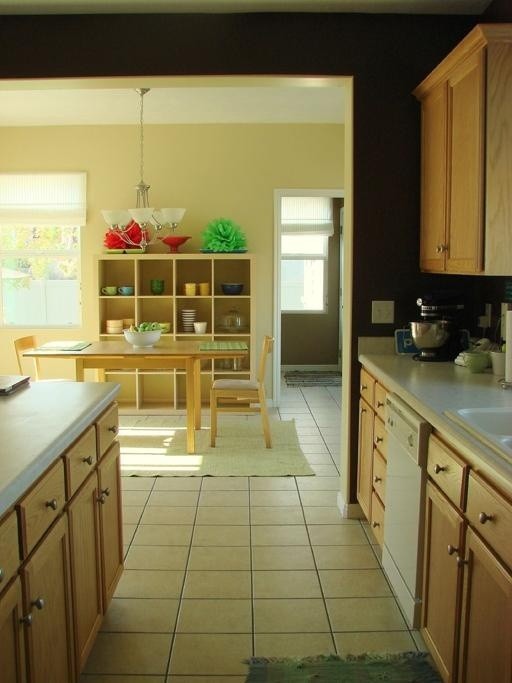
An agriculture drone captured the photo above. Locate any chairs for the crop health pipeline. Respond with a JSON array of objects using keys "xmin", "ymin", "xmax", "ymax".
[
  {"xmin": 210, "ymin": 336, "xmax": 276, "ymax": 450},
  {"xmin": 13, "ymin": 335, "xmax": 75, "ymax": 381}
]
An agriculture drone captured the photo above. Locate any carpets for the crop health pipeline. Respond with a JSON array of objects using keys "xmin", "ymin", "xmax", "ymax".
[
  {"xmin": 242, "ymin": 653, "xmax": 444, "ymax": 683},
  {"xmin": 119, "ymin": 419, "xmax": 316, "ymax": 477},
  {"xmin": 284, "ymin": 371, "xmax": 342, "ymax": 387}
]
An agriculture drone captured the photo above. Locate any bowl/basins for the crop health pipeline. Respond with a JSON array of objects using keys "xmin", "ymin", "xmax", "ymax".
[
  {"xmin": 123, "ymin": 329, "xmax": 161, "ymax": 348},
  {"xmin": 222, "ymin": 282, "xmax": 243, "ymax": 296}
]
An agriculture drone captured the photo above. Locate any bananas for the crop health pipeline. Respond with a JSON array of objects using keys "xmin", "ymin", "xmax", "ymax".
[{"xmin": 127, "ymin": 326, "xmax": 137, "ymax": 333}]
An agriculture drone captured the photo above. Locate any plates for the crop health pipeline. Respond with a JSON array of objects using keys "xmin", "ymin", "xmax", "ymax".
[
  {"xmin": 105, "ymin": 247, "xmax": 145, "ymax": 253},
  {"xmin": 105, "ymin": 319, "xmax": 134, "ymax": 334},
  {"xmin": 199, "ymin": 248, "xmax": 248, "ymax": 252},
  {"xmin": 181, "ymin": 309, "xmax": 198, "ymax": 332}
]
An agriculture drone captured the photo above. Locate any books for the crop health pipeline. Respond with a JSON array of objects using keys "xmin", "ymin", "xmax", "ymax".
[{"xmin": 1, "ymin": 375, "xmax": 31, "ymax": 395}]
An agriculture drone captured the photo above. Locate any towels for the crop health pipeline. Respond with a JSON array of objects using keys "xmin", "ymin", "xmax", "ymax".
[
  {"xmin": 37, "ymin": 342, "xmax": 91, "ymax": 351},
  {"xmin": 199, "ymin": 341, "xmax": 248, "ymax": 350}
]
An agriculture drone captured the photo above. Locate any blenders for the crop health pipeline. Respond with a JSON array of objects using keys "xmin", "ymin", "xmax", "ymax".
[{"xmin": 409, "ymin": 297, "xmax": 470, "ymax": 362}]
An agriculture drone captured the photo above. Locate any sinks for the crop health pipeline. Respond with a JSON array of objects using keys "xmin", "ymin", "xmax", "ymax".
[
  {"xmin": 443, "ymin": 407, "xmax": 512, "ymax": 437},
  {"xmin": 495, "ymin": 439, "xmax": 512, "ymax": 463}
]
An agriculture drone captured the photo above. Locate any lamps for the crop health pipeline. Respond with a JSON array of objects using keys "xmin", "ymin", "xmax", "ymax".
[{"xmin": 100, "ymin": 88, "xmax": 186, "ymax": 252}]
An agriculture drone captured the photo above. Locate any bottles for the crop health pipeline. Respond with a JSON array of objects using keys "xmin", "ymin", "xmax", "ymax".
[{"xmin": 225, "ymin": 305, "xmax": 243, "ymax": 333}]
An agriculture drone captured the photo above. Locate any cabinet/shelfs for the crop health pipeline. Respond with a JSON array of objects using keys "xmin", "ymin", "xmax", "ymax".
[
  {"xmin": 356, "ymin": 366, "xmax": 389, "ymax": 554},
  {"xmin": 418, "ymin": 429, "xmax": 512, "ymax": 683},
  {"xmin": 94, "ymin": 254, "xmax": 256, "ymax": 417},
  {"xmin": 411, "ymin": 23, "xmax": 512, "ymax": 277},
  {"xmin": 0, "ymin": 401, "xmax": 123, "ymax": 683}
]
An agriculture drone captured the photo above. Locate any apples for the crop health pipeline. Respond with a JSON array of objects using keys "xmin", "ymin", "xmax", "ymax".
[{"xmin": 137, "ymin": 322, "xmax": 160, "ymax": 332}]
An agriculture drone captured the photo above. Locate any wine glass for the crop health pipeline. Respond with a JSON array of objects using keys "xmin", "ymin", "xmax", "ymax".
[{"xmin": 157, "ymin": 235, "xmax": 192, "ymax": 253}]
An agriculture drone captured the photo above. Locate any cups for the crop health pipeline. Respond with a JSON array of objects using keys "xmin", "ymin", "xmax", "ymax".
[
  {"xmin": 219, "ymin": 357, "xmax": 242, "ymax": 371},
  {"xmin": 102, "ymin": 286, "xmax": 134, "ymax": 296},
  {"xmin": 464, "ymin": 350, "xmax": 505, "ymax": 376},
  {"xmin": 194, "ymin": 322, "xmax": 207, "ymax": 334},
  {"xmin": 185, "ymin": 282, "xmax": 210, "ymax": 296},
  {"xmin": 151, "ymin": 279, "xmax": 164, "ymax": 295},
  {"xmin": 159, "ymin": 322, "xmax": 170, "ymax": 333}
]
